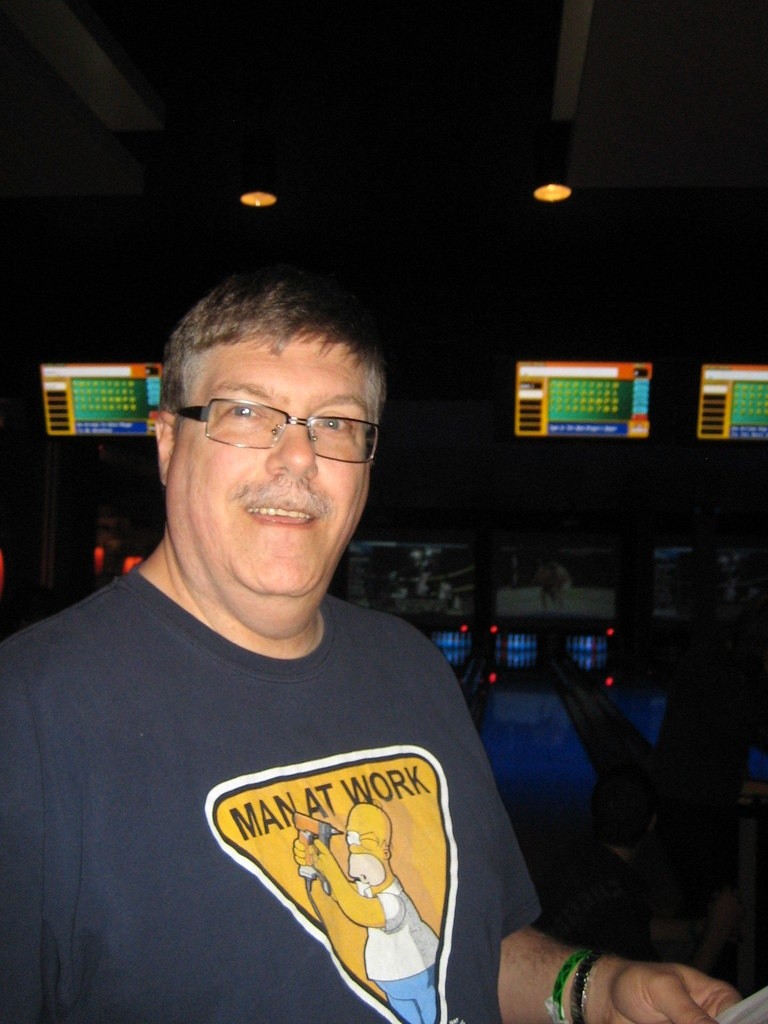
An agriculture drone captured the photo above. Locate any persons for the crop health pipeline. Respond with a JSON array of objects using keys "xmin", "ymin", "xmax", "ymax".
[{"xmin": 0, "ymin": 262, "xmax": 745, "ymax": 1024}]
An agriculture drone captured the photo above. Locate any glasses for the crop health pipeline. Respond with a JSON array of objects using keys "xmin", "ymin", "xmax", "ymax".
[{"xmin": 173, "ymin": 397, "xmax": 379, "ymax": 464}]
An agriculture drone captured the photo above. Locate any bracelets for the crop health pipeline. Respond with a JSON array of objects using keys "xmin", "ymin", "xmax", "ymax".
[{"xmin": 552, "ymin": 948, "xmax": 602, "ymax": 1024}]
